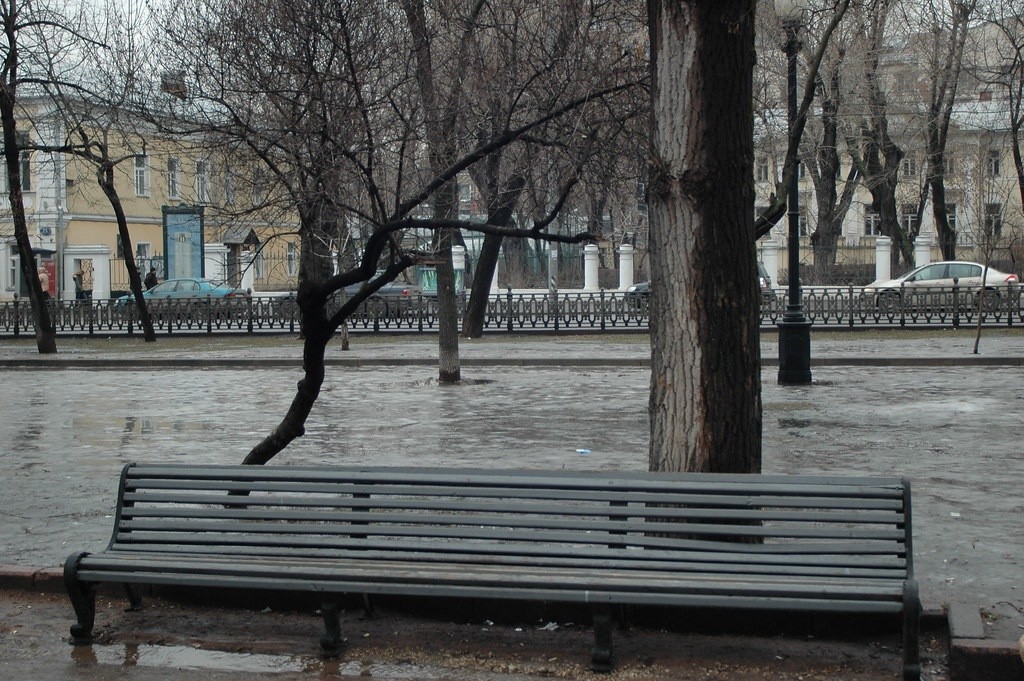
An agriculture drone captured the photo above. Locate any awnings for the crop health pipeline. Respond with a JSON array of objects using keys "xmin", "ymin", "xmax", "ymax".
[{"xmin": 224, "ymin": 227, "xmax": 260, "ymax": 245}]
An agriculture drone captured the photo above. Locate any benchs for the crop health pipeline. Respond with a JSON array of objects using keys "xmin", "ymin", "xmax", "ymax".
[{"xmin": 63, "ymin": 461, "xmax": 922, "ymax": 681}]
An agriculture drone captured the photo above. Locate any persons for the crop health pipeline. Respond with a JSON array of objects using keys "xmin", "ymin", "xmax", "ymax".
[
  {"xmin": 144, "ymin": 267, "xmax": 158, "ymax": 291},
  {"xmin": 73, "ymin": 270, "xmax": 85, "ymax": 299},
  {"xmin": 129, "ymin": 267, "xmax": 142, "ymax": 294},
  {"xmin": 38, "ymin": 267, "xmax": 50, "ymax": 297}
]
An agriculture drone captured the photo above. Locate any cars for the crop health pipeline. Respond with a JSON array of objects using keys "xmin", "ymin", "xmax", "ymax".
[
  {"xmin": 278, "ymin": 274, "xmax": 426, "ymax": 322},
  {"xmin": 114, "ymin": 278, "xmax": 247, "ymax": 312},
  {"xmin": 863, "ymin": 261, "xmax": 1022, "ymax": 311},
  {"xmin": 624, "ymin": 258, "xmax": 776, "ymax": 308}
]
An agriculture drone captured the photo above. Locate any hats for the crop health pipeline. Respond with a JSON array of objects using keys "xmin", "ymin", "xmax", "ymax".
[{"xmin": 151, "ymin": 267, "xmax": 156, "ymax": 272}]
